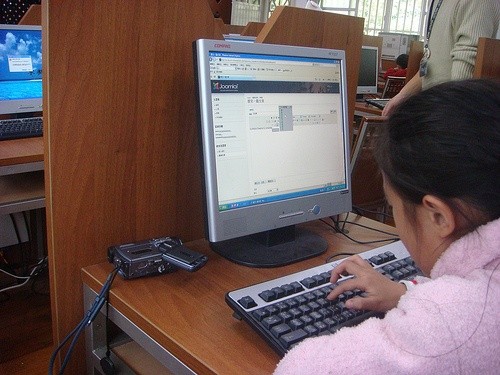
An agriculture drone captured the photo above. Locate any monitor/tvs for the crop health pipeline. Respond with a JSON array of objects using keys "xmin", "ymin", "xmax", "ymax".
[
  {"xmin": 382, "ymin": 77, "xmax": 406, "ymax": 99},
  {"xmin": 0, "ymin": 24, "xmax": 43, "ymax": 118},
  {"xmin": 223, "ymin": 35, "xmax": 257, "ymax": 44},
  {"xmin": 356, "ymin": 45, "xmax": 378, "ymax": 101},
  {"xmin": 192, "ymin": 38, "xmax": 352, "ymax": 267}
]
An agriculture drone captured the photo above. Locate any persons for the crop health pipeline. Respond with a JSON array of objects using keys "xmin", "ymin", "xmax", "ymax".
[
  {"xmin": 382, "ymin": 0, "xmax": 500, "ymax": 116},
  {"xmin": 383, "ymin": 54, "xmax": 409, "ymax": 79},
  {"xmin": 273, "ymin": 79, "xmax": 500, "ymax": 375}
]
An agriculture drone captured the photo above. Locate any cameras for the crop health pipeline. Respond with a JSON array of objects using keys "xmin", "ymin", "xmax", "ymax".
[{"xmin": 106, "ymin": 236, "xmax": 208, "ymax": 281}]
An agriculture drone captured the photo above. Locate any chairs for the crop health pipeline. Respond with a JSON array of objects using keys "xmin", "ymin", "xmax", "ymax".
[
  {"xmin": 378, "ymin": 76, "xmax": 407, "ymax": 100},
  {"xmin": 351, "ymin": 116, "xmax": 391, "ymax": 223}
]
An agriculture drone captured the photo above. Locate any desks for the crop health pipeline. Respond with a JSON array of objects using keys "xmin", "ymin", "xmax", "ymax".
[
  {"xmin": 81, "ymin": 212, "xmax": 401, "ymax": 375},
  {"xmin": 355, "ymin": 102, "xmax": 382, "ymax": 117},
  {"xmin": 0, "ymin": 137, "xmax": 47, "ymax": 243}
]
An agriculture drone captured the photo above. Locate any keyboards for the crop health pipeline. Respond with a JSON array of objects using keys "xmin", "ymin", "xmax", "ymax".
[
  {"xmin": 224, "ymin": 238, "xmax": 429, "ymax": 358},
  {"xmin": 0, "ymin": 117, "xmax": 43, "ymax": 141}
]
